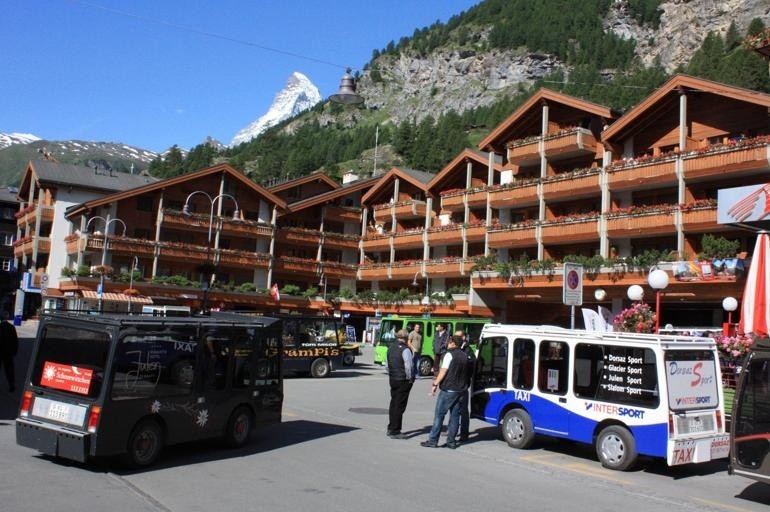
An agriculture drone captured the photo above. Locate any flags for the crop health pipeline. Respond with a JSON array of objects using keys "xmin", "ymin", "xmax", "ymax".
[{"xmin": 269, "ymin": 283, "xmax": 280, "ymax": 309}]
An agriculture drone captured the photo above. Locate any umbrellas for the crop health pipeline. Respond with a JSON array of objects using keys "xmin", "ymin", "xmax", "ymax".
[{"xmin": 738, "ymin": 229, "xmax": 770, "ymax": 340}]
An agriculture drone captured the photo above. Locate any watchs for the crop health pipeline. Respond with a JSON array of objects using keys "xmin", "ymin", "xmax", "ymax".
[{"xmin": 432, "ymin": 383, "xmax": 438, "ymax": 387}]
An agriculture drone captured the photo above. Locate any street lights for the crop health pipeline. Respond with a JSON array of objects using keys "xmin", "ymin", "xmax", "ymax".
[
  {"xmin": 318, "ymin": 272, "xmax": 327, "ymax": 336},
  {"xmin": 128, "ymin": 256, "xmax": 142, "ymax": 313},
  {"xmin": 411, "ymin": 271, "xmax": 429, "ymax": 316},
  {"xmin": 177, "ymin": 192, "xmax": 244, "ymax": 309},
  {"xmin": 627, "ymin": 285, "xmax": 645, "ymax": 333},
  {"xmin": 722, "ymin": 296, "xmax": 738, "ymax": 322},
  {"xmin": 648, "ymin": 269, "xmax": 669, "ymax": 334},
  {"xmin": 81, "ymin": 214, "xmax": 128, "ymax": 315}
]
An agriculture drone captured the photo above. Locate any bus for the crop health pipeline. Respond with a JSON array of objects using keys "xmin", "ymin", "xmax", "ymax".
[
  {"xmin": 372, "ymin": 315, "xmax": 495, "ymax": 377},
  {"xmin": 657, "ymin": 327, "xmax": 724, "ymax": 339},
  {"xmin": 470, "ymin": 323, "xmax": 731, "ymax": 471},
  {"xmin": 727, "ymin": 335, "xmax": 769, "ymax": 485},
  {"xmin": 210, "ymin": 310, "xmax": 343, "ymax": 380},
  {"xmin": 142, "ymin": 305, "xmax": 190, "ymax": 318},
  {"xmin": 365, "ymin": 315, "xmax": 421, "ymax": 343},
  {"xmin": 15, "ymin": 308, "xmax": 284, "ymax": 470}
]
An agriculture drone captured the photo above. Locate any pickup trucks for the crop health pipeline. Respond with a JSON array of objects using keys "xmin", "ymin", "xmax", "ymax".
[{"xmin": 340, "ymin": 342, "xmax": 363, "ymax": 366}]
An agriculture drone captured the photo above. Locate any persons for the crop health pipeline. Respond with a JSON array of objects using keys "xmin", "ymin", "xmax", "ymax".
[
  {"xmin": 431, "ymin": 322, "xmax": 449, "ymax": 379},
  {"xmin": 420, "ymin": 336, "xmax": 470, "ymax": 448},
  {"xmin": 0, "ymin": 310, "xmax": 19, "ymax": 393},
  {"xmin": 407, "ymin": 324, "xmax": 423, "ymax": 380},
  {"xmin": 440, "ymin": 329, "xmax": 476, "ymax": 445},
  {"xmin": 385, "ymin": 329, "xmax": 416, "ymax": 439}
]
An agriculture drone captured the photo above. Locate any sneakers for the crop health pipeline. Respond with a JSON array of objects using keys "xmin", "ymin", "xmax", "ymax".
[
  {"xmin": 441, "ymin": 432, "xmax": 448, "ymax": 436},
  {"xmin": 447, "ymin": 444, "xmax": 456, "ymax": 449},
  {"xmin": 459, "ymin": 436, "xmax": 468, "ymax": 442},
  {"xmin": 390, "ymin": 433, "xmax": 408, "ymax": 439},
  {"xmin": 421, "ymin": 441, "xmax": 437, "ymax": 448}
]
